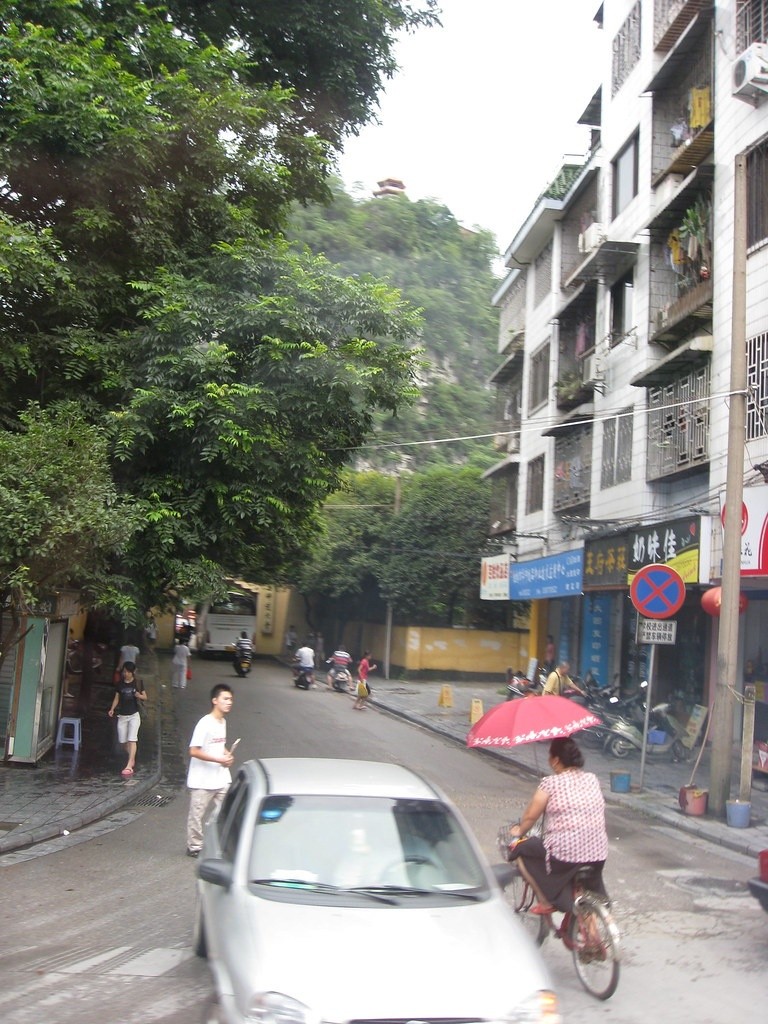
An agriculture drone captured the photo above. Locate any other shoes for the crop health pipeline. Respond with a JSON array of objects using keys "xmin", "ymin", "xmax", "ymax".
[
  {"xmin": 359, "ymin": 706, "xmax": 367, "ymax": 710},
  {"xmin": 353, "ymin": 706, "xmax": 362, "ymax": 711},
  {"xmin": 63, "ymin": 693, "xmax": 75, "ymax": 698},
  {"xmin": 350, "ymin": 686, "xmax": 355, "ymax": 689},
  {"xmin": 186, "ymin": 848, "xmax": 200, "ymax": 858}
]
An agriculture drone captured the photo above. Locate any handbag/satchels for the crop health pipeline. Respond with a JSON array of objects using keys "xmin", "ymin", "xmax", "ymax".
[
  {"xmin": 135, "ymin": 679, "xmax": 147, "ymax": 720},
  {"xmin": 114, "ymin": 673, "xmax": 120, "ymax": 685},
  {"xmin": 187, "ymin": 668, "xmax": 192, "ymax": 679},
  {"xmin": 355, "ymin": 679, "xmax": 371, "ymax": 697}
]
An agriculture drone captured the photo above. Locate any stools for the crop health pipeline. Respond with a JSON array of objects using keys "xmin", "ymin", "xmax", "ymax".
[{"xmin": 56, "ymin": 716, "xmax": 82, "ymax": 751}]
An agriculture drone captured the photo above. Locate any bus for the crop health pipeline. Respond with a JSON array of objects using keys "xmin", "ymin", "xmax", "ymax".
[{"xmin": 192, "ymin": 589, "xmax": 258, "ymax": 660}]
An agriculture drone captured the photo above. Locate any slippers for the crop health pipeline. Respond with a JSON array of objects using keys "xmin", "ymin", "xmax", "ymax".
[
  {"xmin": 530, "ymin": 903, "xmax": 557, "ymax": 916},
  {"xmin": 122, "ymin": 767, "xmax": 134, "ymax": 774}
]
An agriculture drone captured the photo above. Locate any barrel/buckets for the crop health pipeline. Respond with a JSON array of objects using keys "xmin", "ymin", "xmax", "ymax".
[
  {"xmin": 609, "ymin": 769, "xmax": 631, "ymax": 792},
  {"xmin": 726, "ymin": 800, "xmax": 751, "ymax": 827},
  {"xmin": 683, "ymin": 789, "xmax": 709, "ymax": 815}
]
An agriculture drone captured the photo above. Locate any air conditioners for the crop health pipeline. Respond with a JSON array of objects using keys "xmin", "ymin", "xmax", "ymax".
[{"xmin": 730, "ymin": 41, "xmax": 768, "ymax": 94}]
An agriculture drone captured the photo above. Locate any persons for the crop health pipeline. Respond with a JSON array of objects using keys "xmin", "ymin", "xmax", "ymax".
[
  {"xmin": 326, "ymin": 644, "xmax": 356, "ymax": 689},
  {"xmin": 146, "ymin": 617, "xmax": 158, "ymax": 647},
  {"xmin": 186, "ymin": 682, "xmax": 234, "ymax": 858},
  {"xmin": 118, "ymin": 638, "xmax": 140, "ymax": 676},
  {"xmin": 171, "ymin": 640, "xmax": 191, "ymax": 690},
  {"xmin": 509, "ymin": 736, "xmax": 614, "ymax": 939},
  {"xmin": 109, "ymin": 661, "xmax": 146, "ymax": 774},
  {"xmin": 285, "ymin": 625, "xmax": 297, "ymax": 661},
  {"xmin": 541, "ymin": 660, "xmax": 589, "ymax": 700},
  {"xmin": 350, "ymin": 649, "xmax": 378, "ymax": 710},
  {"xmin": 234, "ymin": 631, "xmax": 256, "ymax": 660},
  {"xmin": 544, "ymin": 635, "xmax": 556, "ymax": 666},
  {"xmin": 291, "ymin": 642, "xmax": 317, "ymax": 690}
]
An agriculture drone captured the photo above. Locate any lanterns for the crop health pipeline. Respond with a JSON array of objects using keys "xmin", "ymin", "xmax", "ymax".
[{"xmin": 701, "ymin": 585, "xmax": 747, "ymax": 618}]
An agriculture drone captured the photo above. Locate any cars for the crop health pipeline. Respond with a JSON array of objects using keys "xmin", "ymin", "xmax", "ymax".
[
  {"xmin": 176, "ymin": 617, "xmax": 192, "ymax": 646},
  {"xmin": 195, "ymin": 757, "xmax": 562, "ymax": 1024}
]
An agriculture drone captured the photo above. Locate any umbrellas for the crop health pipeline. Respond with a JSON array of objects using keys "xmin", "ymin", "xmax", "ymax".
[{"xmin": 463, "ymin": 694, "xmax": 604, "ymax": 780}]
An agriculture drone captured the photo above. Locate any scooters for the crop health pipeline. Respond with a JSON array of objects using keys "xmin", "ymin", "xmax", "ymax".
[
  {"xmin": 327, "ymin": 659, "xmax": 351, "ymax": 692},
  {"xmin": 506, "ymin": 660, "xmax": 693, "ymax": 760},
  {"xmin": 291, "ymin": 654, "xmax": 316, "ymax": 691},
  {"xmin": 231, "ymin": 643, "xmax": 256, "ymax": 677}
]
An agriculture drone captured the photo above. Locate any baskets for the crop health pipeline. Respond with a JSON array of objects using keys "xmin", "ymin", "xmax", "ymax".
[{"xmin": 498, "ymin": 821, "xmax": 544, "ymax": 862}]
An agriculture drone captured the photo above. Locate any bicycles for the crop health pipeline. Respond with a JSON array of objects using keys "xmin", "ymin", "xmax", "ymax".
[{"xmin": 496, "ymin": 735, "xmax": 624, "ymax": 1003}]
